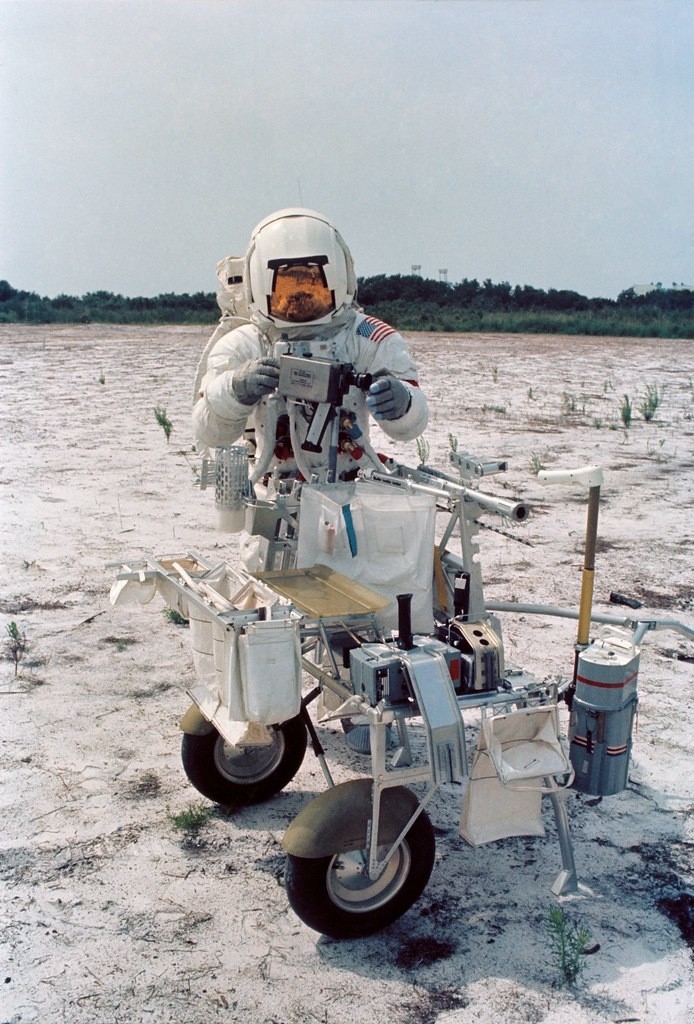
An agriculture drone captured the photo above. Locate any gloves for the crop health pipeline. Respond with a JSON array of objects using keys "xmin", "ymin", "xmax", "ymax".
[
  {"xmin": 365, "ymin": 375, "xmax": 411, "ymax": 421},
  {"xmin": 233, "ymin": 353, "xmax": 281, "ymax": 406}
]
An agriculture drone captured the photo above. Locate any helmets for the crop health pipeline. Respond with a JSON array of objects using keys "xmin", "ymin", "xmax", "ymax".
[{"xmin": 243, "ymin": 208, "xmax": 359, "ymax": 336}]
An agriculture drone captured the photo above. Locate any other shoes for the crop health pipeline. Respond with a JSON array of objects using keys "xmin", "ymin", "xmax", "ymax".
[{"xmin": 346, "ymin": 724, "xmax": 390, "ymax": 755}]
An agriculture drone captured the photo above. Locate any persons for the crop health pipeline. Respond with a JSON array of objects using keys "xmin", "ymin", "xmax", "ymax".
[{"xmin": 190, "ymin": 207, "xmax": 429, "ymax": 753}]
